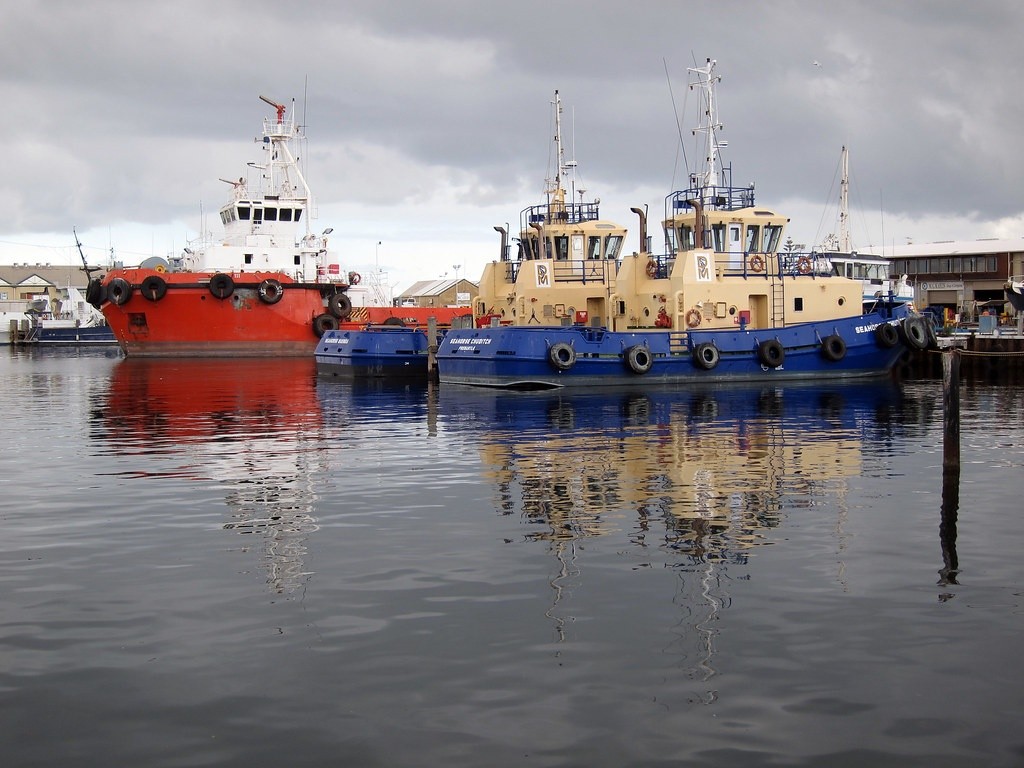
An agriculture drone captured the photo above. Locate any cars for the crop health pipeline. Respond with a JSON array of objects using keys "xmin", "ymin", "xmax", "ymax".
[{"xmin": 395, "ymin": 298, "xmax": 417, "ymax": 306}]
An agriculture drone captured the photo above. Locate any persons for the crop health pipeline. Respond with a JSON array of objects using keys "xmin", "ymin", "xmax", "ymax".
[{"xmin": 982, "ymin": 309, "xmax": 990, "ymax": 316}]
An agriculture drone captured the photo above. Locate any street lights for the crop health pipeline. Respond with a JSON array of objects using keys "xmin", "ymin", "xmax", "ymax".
[{"xmin": 452, "ymin": 265, "xmax": 461, "ymax": 308}]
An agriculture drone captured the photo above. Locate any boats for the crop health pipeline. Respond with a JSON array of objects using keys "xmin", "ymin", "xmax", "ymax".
[
  {"xmin": 313, "ymin": 329, "xmax": 447, "ymax": 377},
  {"xmin": 433, "ymin": 47, "xmax": 938, "ymax": 388},
  {"xmin": 936, "ymin": 299, "xmax": 1024, "ymax": 356},
  {"xmin": 84, "ymin": 74, "xmax": 362, "ymax": 356},
  {"xmin": 23, "ymin": 290, "xmax": 118, "ymax": 346}
]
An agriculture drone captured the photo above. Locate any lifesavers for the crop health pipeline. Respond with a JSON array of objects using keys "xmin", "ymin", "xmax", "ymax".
[
  {"xmin": 823, "ymin": 335, "xmax": 847, "ymax": 361},
  {"xmin": 693, "ymin": 342, "xmax": 720, "ymax": 369},
  {"xmin": 875, "ymin": 323, "xmax": 898, "ymax": 346},
  {"xmin": 646, "ymin": 260, "xmax": 657, "ymax": 276},
  {"xmin": 209, "ymin": 274, "xmax": 235, "ymax": 299},
  {"xmin": 750, "ymin": 255, "xmax": 765, "ymax": 272},
  {"xmin": 313, "ymin": 313, "xmax": 339, "ymax": 338},
  {"xmin": 108, "ymin": 277, "xmax": 132, "ymax": 305},
  {"xmin": 258, "ymin": 278, "xmax": 284, "ymax": 304},
  {"xmin": 330, "ymin": 293, "xmax": 353, "ymax": 319},
  {"xmin": 86, "ymin": 277, "xmax": 100, "ymax": 301},
  {"xmin": 549, "ymin": 342, "xmax": 576, "ymax": 369},
  {"xmin": 901, "ymin": 318, "xmax": 929, "ymax": 350},
  {"xmin": 798, "ymin": 257, "xmax": 812, "ymax": 274},
  {"xmin": 686, "ymin": 309, "xmax": 702, "ymax": 327},
  {"xmin": 140, "ymin": 275, "xmax": 167, "ymax": 301},
  {"xmin": 625, "ymin": 345, "xmax": 652, "ymax": 373},
  {"xmin": 384, "ymin": 317, "xmax": 405, "ymax": 327},
  {"xmin": 759, "ymin": 339, "xmax": 784, "ymax": 367}
]
